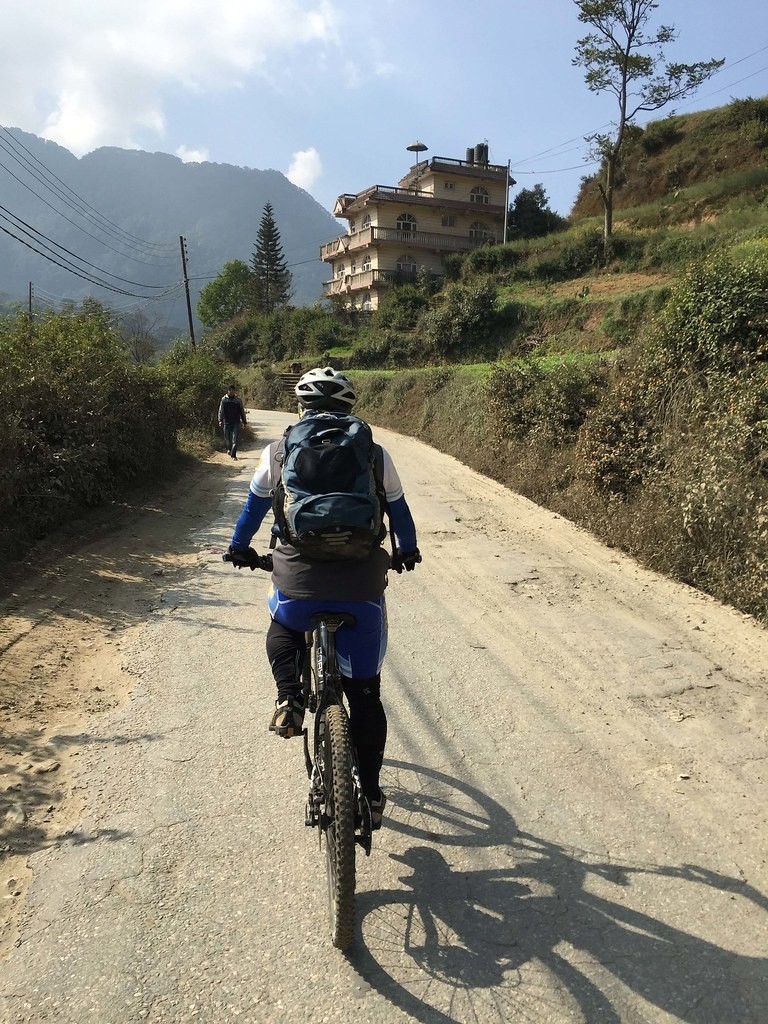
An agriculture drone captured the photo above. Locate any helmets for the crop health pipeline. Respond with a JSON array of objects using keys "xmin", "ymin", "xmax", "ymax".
[{"xmin": 295, "ymin": 366, "xmax": 357, "ymax": 414}]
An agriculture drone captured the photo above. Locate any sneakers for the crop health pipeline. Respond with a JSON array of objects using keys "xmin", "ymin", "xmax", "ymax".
[
  {"xmin": 369, "ymin": 788, "xmax": 387, "ymax": 829},
  {"xmin": 268, "ymin": 695, "xmax": 305, "ymax": 735}
]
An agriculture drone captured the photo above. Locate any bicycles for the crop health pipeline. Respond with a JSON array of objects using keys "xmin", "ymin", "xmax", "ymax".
[{"xmin": 222, "ymin": 550, "xmax": 422, "ymax": 951}]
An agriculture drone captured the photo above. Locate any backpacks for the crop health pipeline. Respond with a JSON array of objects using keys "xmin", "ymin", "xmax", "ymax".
[{"xmin": 270, "ymin": 412, "xmax": 389, "ymax": 566}]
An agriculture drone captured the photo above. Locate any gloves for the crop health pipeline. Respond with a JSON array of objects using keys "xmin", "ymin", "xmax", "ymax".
[
  {"xmin": 229, "ymin": 545, "xmax": 260, "ymax": 571},
  {"xmin": 392, "ymin": 547, "xmax": 420, "ymax": 574}
]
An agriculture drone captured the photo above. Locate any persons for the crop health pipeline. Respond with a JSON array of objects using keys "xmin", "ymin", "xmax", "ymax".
[
  {"xmin": 218, "ymin": 385, "xmax": 246, "ymax": 460},
  {"xmin": 226, "ymin": 368, "xmax": 419, "ymax": 829}
]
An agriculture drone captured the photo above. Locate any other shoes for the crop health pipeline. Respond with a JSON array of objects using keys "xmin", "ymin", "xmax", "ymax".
[
  {"xmin": 232, "ymin": 457, "xmax": 237, "ymax": 461},
  {"xmin": 228, "ymin": 450, "xmax": 231, "ymax": 455}
]
